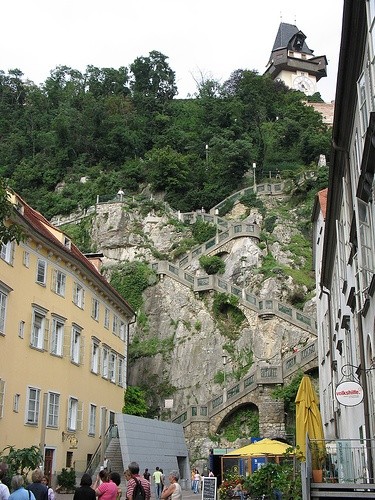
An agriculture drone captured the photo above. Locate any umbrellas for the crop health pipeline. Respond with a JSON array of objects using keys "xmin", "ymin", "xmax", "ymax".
[
  {"xmin": 240, "ymin": 440, "xmax": 300, "ymax": 464},
  {"xmin": 295, "ymin": 374, "xmax": 326, "ymax": 462},
  {"xmin": 225, "ymin": 438, "xmax": 295, "ymax": 465}
]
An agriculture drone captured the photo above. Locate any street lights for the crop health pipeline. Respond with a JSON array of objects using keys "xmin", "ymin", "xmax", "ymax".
[
  {"xmin": 252, "ymin": 162, "xmax": 258, "ymax": 194},
  {"xmin": 214, "ymin": 208, "xmax": 221, "ymax": 245},
  {"xmin": 205, "ymin": 144, "xmax": 208, "ymax": 163}
]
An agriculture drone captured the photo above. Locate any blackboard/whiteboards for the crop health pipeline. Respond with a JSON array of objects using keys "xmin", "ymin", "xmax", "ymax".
[{"xmin": 202, "ymin": 476, "xmax": 217, "ymax": 499}]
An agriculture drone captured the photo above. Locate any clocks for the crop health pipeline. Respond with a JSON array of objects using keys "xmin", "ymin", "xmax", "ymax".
[{"xmin": 293, "ymin": 76, "xmax": 315, "ymax": 96}]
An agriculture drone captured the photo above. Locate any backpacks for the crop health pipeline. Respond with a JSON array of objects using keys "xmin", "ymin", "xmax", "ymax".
[{"xmin": 133, "ymin": 477, "xmax": 146, "ymax": 500}]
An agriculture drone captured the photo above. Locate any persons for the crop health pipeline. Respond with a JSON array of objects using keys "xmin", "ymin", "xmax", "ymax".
[
  {"xmin": 191, "ymin": 467, "xmax": 201, "ymax": 494},
  {"xmin": 153, "ymin": 467, "xmax": 165, "ymax": 500},
  {"xmin": 201, "ymin": 464, "xmax": 214, "ymax": 477},
  {"xmin": 292, "ymin": 347, "xmax": 299, "ymax": 353},
  {"xmin": 73, "ymin": 470, "xmax": 122, "ymax": 500},
  {"xmin": 104, "ymin": 456, "xmax": 109, "ymax": 471},
  {"xmin": 124, "ymin": 462, "xmax": 151, "ymax": 500},
  {"xmin": 232, "ymin": 479, "xmax": 245, "ymax": 500},
  {"xmin": 200, "ymin": 473, "xmax": 204, "ymax": 491},
  {"xmin": 100, "ymin": 464, "xmax": 104, "ymax": 471},
  {"xmin": 161, "ymin": 471, "xmax": 183, "ymax": 500},
  {"xmin": 0, "ymin": 461, "xmax": 56, "ymax": 500}
]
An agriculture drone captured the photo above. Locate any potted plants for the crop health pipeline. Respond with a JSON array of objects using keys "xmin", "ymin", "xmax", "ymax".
[
  {"xmin": 309, "ymin": 442, "xmax": 323, "ymax": 483},
  {"xmin": 57, "ymin": 468, "xmax": 77, "ymax": 493}
]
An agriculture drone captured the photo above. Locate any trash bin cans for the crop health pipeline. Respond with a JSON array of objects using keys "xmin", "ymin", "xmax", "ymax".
[{"xmin": 181, "ymin": 479, "xmax": 187, "ymax": 489}]
